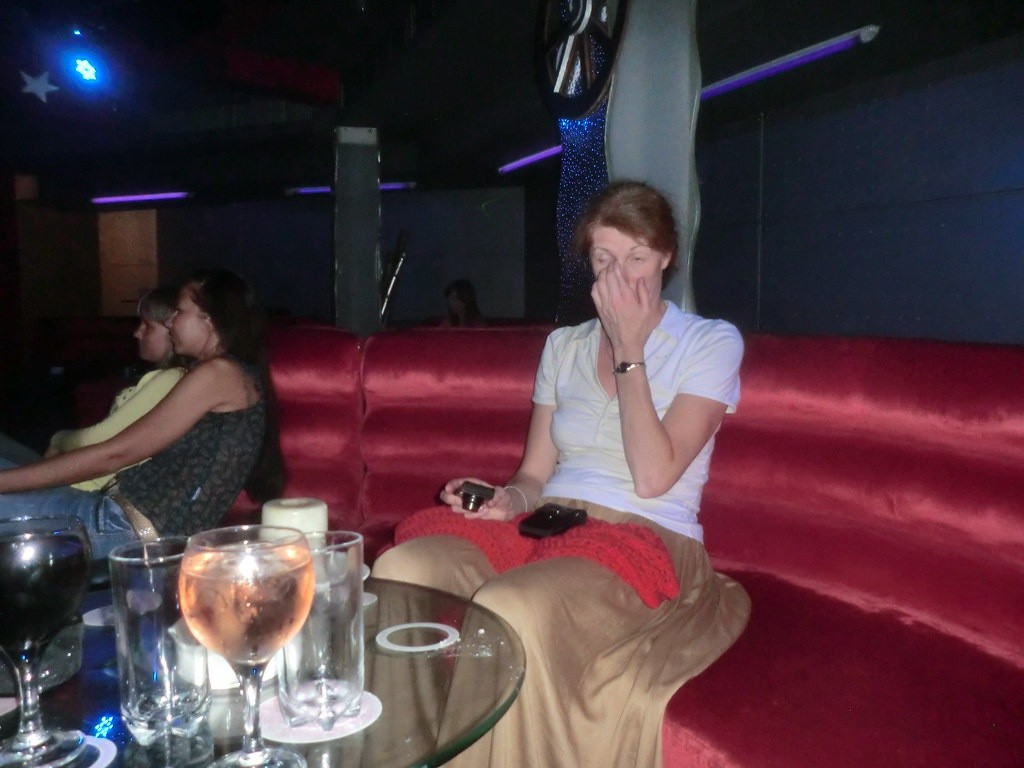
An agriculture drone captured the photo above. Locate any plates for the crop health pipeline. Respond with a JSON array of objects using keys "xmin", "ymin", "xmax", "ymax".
[{"xmin": 314, "ymin": 554, "xmax": 370, "ymax": 594}]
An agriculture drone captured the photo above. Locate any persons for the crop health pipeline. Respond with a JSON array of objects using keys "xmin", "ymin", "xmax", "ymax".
[
  {"xmin": 442, "ymin": 279, "xmax": 485, "ymax": 327},
  {"xmin": 307, "ymin": 180, "xmax": 750, "ymax": 768},
  {"xmin": 0, "ymin": 269, "xmax": 287, "ymax": 571}
]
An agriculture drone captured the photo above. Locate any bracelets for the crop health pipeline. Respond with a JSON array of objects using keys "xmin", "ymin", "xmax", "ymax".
[{"xmin": 504, "ymin": 486, "xmax": 527, "ymax": 513}]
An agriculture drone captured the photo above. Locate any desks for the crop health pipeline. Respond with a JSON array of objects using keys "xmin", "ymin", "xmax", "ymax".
[{"xmin": 40, "ymin": 573, "xmax": 530, "ymax": 768}]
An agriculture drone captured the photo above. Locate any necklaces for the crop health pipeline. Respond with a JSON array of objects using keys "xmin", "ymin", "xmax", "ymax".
[{"xmin": 605, "ymin": 337, "xmax": 614, "ymax": 360}]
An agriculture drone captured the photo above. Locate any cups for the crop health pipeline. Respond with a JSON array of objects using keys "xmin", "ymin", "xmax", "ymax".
[
  {"xmin": 276, "ymin": 531, "xmax": 366, "ymax": 728},
  {"xmin": 1, "ymin": 611, "xmax": 86, "ymax": 695},
  {"xmin": 107, "ymin": 535, "xmax": 211, "ymax": 748}
]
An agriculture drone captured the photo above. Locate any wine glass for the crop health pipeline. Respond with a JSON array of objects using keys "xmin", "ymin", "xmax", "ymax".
[
  {"xmin": 0, "ymin": 515, "xmax": 91, "ymax": 768},
  {"xmin": 178, "ymin": 524, "xmax": 316, "ymax": 768}
]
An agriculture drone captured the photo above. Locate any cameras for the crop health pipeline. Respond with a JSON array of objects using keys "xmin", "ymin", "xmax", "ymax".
[{"xmin": 453, "ymin": 480, "xmax": 495, "ymax": 513}]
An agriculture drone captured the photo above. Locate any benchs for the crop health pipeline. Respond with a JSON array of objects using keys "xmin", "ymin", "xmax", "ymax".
[{"xmin": 67, "ymin": 317, "xmax": 1024, "ymax": 767}]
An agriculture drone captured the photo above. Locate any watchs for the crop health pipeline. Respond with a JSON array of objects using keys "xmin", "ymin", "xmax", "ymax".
[{"xmin": 613, "ymin": 362, "xmax": 646, "ymax": 374}]
